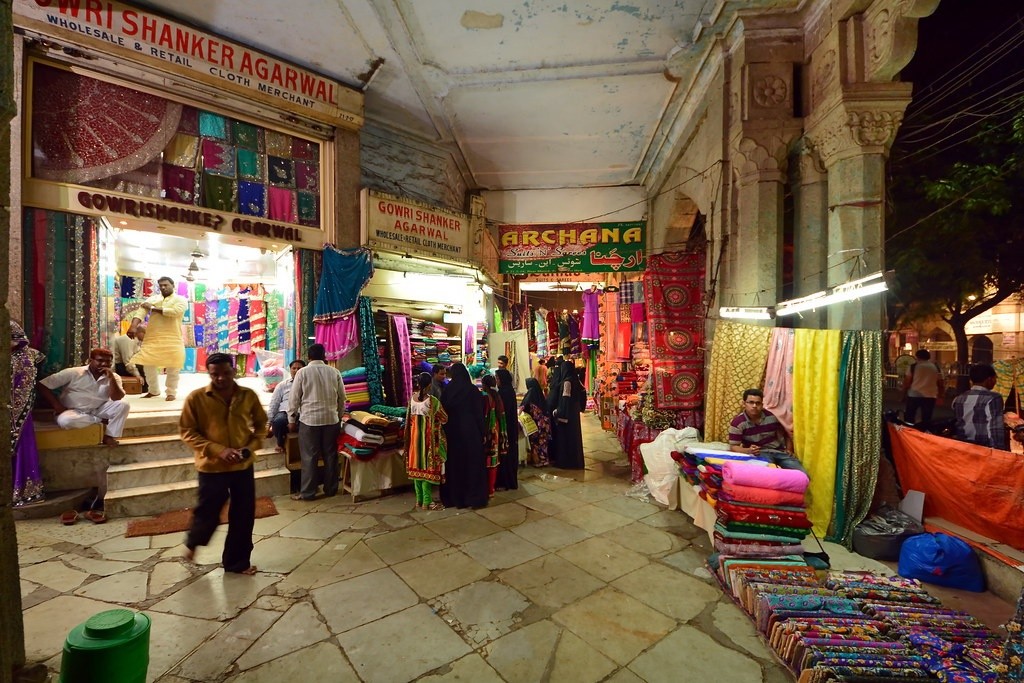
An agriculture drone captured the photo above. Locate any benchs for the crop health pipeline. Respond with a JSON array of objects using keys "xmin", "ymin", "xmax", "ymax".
[
  {"xmin": 32, "ymin": 423, "xmax": 105, "ymax": 449},
  {"xmin": 121, "ymin": 376, "xmax": 142, "ymax": 394}
]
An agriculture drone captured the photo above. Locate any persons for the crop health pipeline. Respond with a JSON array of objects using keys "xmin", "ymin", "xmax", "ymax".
[
  {"xmin": 38, "ymin": 349, "xmax": 129, "ymax": 447},
  {"xmin": 950, "ymin": 364, "xmax": 1006, "ymax": 450},
  {"xmin": 114, "ymin": 277, "xmax": 188, "ymax": 401},
  {"xmin": 729, "ymin": 389, "xmax": 811, "ymax": 480},
  {"xmin": 9, "ymin": 320, "xmax": 48, "ymax": 507},
  {"xmin": 178, "ymin": 353, "xmax": 268, "ymax": 576},
  {"xmin": 402, "ymin": 372, "xmax": 448, "ymax": 511},
  {"xmin": 897, "ymin": 349, "xmax": 945, "ymax": 431},
  {"xmin": 427, "ymin": 355, "xmax": 586, "ymax": 509},
  {"xmin": 266, "ymin": 344, "xmax": 346, "ymax": 500}
]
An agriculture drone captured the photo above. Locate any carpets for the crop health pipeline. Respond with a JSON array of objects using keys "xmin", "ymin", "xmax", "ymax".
[{"xmin": 126, "ymin": 496, "xmax": 279, "ymax": 538}]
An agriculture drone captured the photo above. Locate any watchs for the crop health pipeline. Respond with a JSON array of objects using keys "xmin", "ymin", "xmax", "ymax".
[{"xmin": 150, "ymin": 305, "xmax": 156, "ymax": 312}]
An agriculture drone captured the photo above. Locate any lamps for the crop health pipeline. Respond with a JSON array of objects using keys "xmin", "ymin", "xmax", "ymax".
[
  {"xmin": 185, "ymin": 240, "xmax": 204, "ymax": 280},
  {"xmin": 482, "ymin": 284, "xmax": 493, "ymax": 295},
  {"xmin": 404, "ymin": 271, "xmax": 477, "ymax": 283},
  {"xmin": 719, "ymin": 268, "xmax": 896, "ymax": 321}
]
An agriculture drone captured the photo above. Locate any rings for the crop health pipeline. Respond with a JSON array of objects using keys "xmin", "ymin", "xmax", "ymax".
[
  {"xmin": 753, "ymin": 448, "xmax": 754, "ymax": 451},
  {"xmin": 231, "ymin": 454, "xmax": 235, "ymax": 457}
]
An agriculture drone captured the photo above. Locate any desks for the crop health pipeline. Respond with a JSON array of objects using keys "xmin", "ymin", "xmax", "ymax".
[
  {"xmin": 339, "ymin": 428, "xmax": 531, "ymax": 503},
  {"xmin": 600, "ymin": 395, "xmax": 718, "ymax": 550}
]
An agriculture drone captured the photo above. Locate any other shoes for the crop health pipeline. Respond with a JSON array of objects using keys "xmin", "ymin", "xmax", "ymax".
[
  {"xmin": 84, "ymin": 510, "xmax": 106, "ymax": 522},
  {"xmin": 60, "ymin": 509, "xmax": 78, "ymax": 524},
  {"xmin": 423, "ymin": 503, "xmax": 443, "ymax": 510},
  {"xmin": 416, "ymin": 502, "xmax": 422, "ymax": 507}
]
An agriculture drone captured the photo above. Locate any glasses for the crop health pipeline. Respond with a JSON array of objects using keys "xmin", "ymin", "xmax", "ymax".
[
  {"xmin": 746, "ymin": 401, "xmax": 762, "ymax": 406},
  {"xmin": 91, "ymin": 357, "xmax": 114, "ymax": 365}
]
